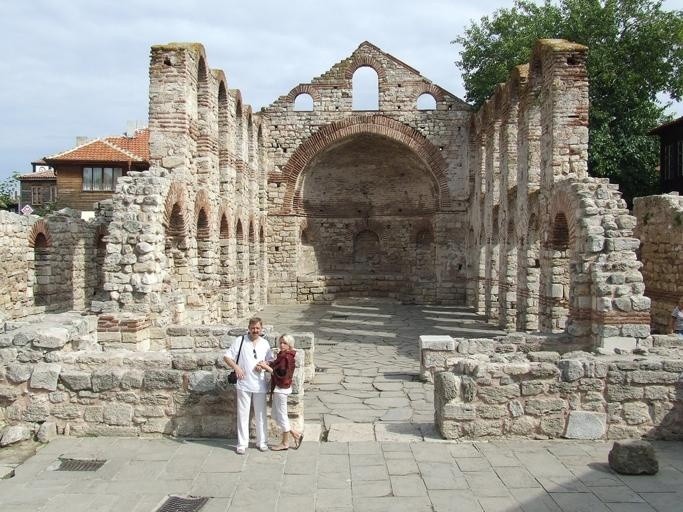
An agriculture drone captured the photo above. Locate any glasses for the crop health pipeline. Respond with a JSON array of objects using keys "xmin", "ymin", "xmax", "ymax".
[{"xmin": 253, "ymin": 348, "xmax": 257, "ymax": 360}]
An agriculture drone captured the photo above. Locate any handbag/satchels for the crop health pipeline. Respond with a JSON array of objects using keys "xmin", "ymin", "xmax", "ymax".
[{"xmin": 227, "ymin": 369, "xmax": 237, "ymax": 385}]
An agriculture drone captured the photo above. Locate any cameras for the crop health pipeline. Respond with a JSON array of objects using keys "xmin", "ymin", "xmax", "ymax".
[{"xmin": 228, "ymin": 372, "xmax": 236, "ymax": 384}]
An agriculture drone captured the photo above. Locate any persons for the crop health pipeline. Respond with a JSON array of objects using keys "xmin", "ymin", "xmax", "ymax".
[
  {"xmin": 669, "ymin": 296, "xmax": 683, "ymax": 335},
  {"xmin": 223, "ymin": 317, "xmax": 275, "ymax": 455},
  {"xmin": 257, "ymin": 332, "xmax": 304, "ymax": 451}
]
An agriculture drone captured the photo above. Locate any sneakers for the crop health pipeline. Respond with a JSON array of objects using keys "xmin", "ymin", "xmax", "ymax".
[
  {"xmin": 295, "ymin": 432, "xmax": 304, "ymax": 449},
  {"xmin": 270, "ymin": 440, "xmax": 289, "ymax": 451},
  {"xmin": 236, "ymin": 445, "xmax": 268, "ymax": 454}
]
